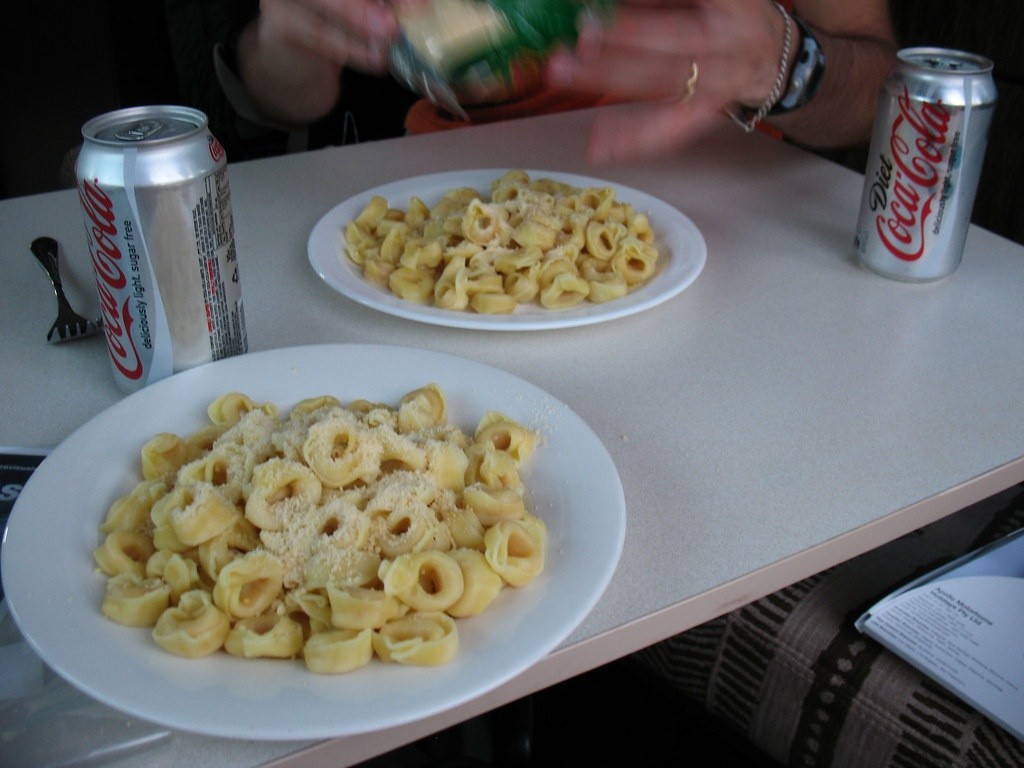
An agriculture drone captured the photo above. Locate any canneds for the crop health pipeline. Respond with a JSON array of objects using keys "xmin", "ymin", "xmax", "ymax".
[
  {"xmin": 74, "ymin": 105, "xmax": 250, "ymax": 393},
  {"xmin": 853, "ymin": 47, "xmax": 997, "ymax": 285}
]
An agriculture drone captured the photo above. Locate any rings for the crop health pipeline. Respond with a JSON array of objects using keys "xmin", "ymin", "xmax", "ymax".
[{"xmin": 684, "ymin": 62, "xmax": 698, "ymax": 102}]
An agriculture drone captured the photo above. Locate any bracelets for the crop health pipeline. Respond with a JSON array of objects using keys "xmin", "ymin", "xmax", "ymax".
[{"xmin": 725, "ymin": 0, "xmax": 792, "ymax": 134}]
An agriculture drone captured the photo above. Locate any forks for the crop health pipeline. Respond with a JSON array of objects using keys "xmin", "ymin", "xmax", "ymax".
[{"xmin": 30, "ymin": 235, "xmax": 102, "ymax": 342}]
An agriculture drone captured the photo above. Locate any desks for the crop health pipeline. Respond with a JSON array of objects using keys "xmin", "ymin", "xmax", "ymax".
[{"xmin": 0, "ymin": 98, "xmax": 1024, "ymax": 768}]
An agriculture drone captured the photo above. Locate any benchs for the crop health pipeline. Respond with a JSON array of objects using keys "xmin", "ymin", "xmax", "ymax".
[{"xmin": 624, "ymin": 0, "xmax": 1024, "ymax": 768}]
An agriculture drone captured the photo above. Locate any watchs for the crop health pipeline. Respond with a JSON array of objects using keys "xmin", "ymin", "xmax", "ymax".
[{"xmin": 741, "ymin": 13, "xmax": 826, "ymax": 117}]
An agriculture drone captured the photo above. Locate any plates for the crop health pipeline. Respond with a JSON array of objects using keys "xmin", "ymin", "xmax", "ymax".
[
  {"xmin": 307, "ymin": 166, "xmax": 708, "ymax": 331},
  {"xmin": 1, "ymin": 345, "xmax": 628, "ymax": 741}
]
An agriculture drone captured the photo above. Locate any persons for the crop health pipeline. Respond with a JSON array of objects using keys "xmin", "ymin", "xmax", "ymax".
[{"xmin": 1, "ymin": 0, "xmax": 899, "ymax": 190}]
